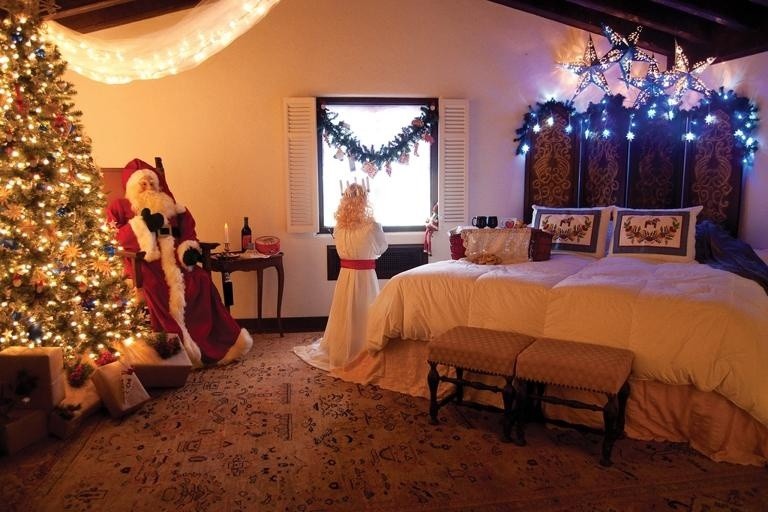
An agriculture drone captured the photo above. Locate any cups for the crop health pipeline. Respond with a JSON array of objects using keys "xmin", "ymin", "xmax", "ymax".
[
  {"xmin": 502, "ymin": 217, "xmax": 517, "ymax": 229},
  {"xmin": 473, "ymin": 215, "xmax": 499, "ymax": 229}
]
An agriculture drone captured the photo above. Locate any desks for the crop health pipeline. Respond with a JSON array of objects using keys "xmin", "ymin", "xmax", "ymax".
[
  {"xmin": 203, "ymin": 251, "xmax": 285, "ymax": 339},
  {"xmin": 448, "ymin": 225, "xmax": 553, "ymax": 261}
]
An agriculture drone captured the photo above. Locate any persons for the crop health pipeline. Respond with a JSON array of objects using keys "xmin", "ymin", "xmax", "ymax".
[
  {"xmin": 292, "ymin": 182, "xmax": 389, "ymax": 375},
  {"xmin": 107, "ymin": 157, "xmax": 254, "ymax": 367},
  {"xmin": 423, "ymin": 202, "xmax": 438, "ymax": 257}
]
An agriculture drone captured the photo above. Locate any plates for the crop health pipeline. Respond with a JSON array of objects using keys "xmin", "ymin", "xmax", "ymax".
[{"xmin": 214, "ymin": 255, "xmax": 240, "ymax": 259}]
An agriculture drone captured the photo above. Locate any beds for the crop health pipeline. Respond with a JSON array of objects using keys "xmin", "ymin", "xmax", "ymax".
[{"xmin": 328, "ymin": 221, "xmax": 768, "ymax": 468}]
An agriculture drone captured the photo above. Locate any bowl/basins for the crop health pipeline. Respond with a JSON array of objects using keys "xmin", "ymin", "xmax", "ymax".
[{"xmin": 255, "ymin": 235, "xmax": 280, "ymax": 255}]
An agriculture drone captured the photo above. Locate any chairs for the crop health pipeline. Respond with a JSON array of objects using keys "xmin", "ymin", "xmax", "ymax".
[{"xmin": 99, "ymin": 155, "xmax": 220, "ymax": 276}]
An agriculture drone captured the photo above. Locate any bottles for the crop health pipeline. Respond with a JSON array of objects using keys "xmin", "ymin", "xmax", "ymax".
[{"xmin": 240, "ymin": 216, "xmax": 253, "ymax": 252}]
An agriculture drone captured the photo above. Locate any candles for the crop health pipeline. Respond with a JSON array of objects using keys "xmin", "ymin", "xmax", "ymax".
[{"xmin": 223, "ymin": 222, "xmax": 229, "ymax": 242}]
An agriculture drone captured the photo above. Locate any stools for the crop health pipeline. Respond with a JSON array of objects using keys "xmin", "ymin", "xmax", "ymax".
[
  {"xmin": 513, "ymin": 336, "xmax": 637, "ymax": 465},
  {"xmin": 426, "ymin": 326, "xmax": 539, "ymax": 441}
]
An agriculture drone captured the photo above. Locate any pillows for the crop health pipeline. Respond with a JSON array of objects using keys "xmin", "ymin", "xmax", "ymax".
[
  {"xmin": 607, "ymin": 205, "xmax": 703, "ymax": 266},
  {"xmin": 530, "ymin": 204, "xmax": 615, "ymax": 261}
]
